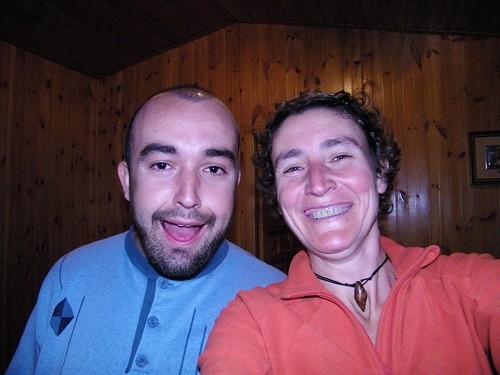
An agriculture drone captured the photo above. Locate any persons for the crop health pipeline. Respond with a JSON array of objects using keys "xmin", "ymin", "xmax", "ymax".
[
  {"xmin": 4, "ymin": 84, "xmax": 288, "ymax": 375},
  {"xmin": 197, "ymin": 90, "xmax": 500, "ymax": 375}
]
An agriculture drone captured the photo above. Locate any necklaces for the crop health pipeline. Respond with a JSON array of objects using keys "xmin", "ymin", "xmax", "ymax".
[{"xmin": 313, "ymin": 253, "xmax": 389, "ymax": 312}]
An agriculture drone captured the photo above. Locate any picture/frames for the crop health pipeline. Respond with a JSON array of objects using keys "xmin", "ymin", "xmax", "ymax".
[{"xmin": 467, "ymin": 130, "xmax": 500, "ymax": 185}]
